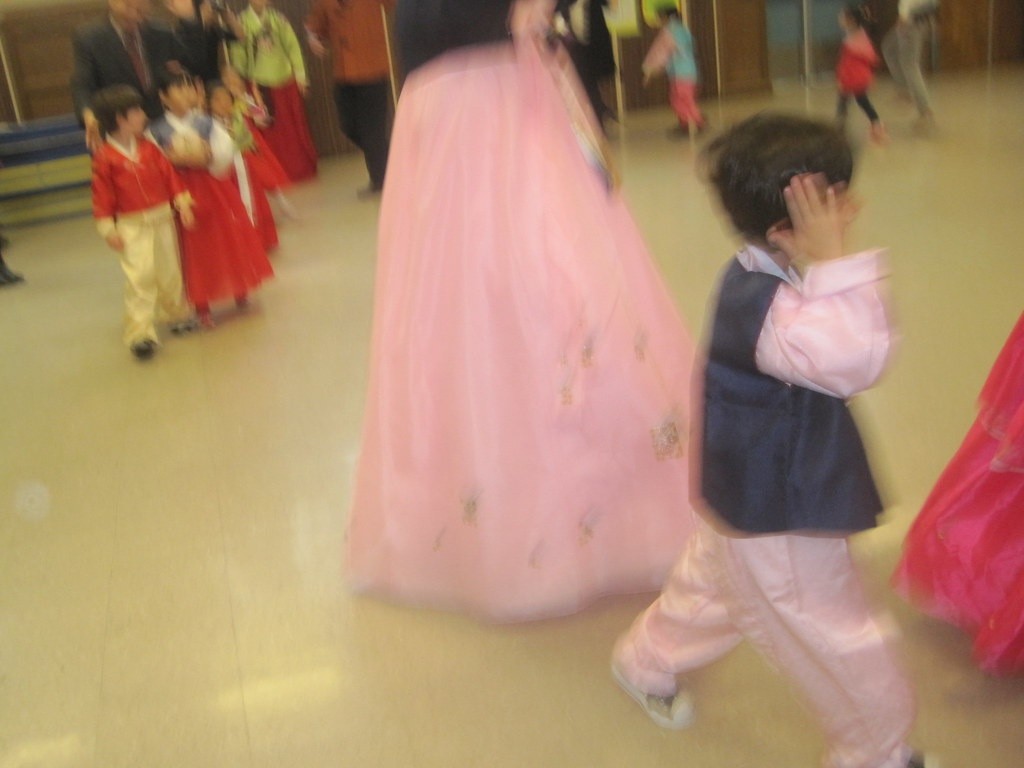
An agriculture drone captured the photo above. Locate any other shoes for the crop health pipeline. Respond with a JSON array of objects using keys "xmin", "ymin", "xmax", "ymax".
[
  {"xmin": 610, "ymin": 649, "xmax": 694, "ymax": 727},
  {"xmin": 170, "ymin": 320, "xmax": 195, "ymax": 332},
  {"xmin": 130, "ymin": 337, "xmax": 157, "ymax": 359},
  {"xmin": 697, "ymin": 121, "xmax": 712, "ymax": 136},
  {"xmin": 668, "ymin": 117, "xmax": 690, "ymax": 140},
  {"xmin": 904, "ymin": 746, "xmax": 939, "ymax": 768}
]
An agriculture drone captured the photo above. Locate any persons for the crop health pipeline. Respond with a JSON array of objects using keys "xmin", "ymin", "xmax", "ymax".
[
  {"xmin": 86, "ymin": 85, "xmax": 197, "ymax": 355},
  {"xmin": 610, "ymin": 115, "xmax": 940, "ymax": 768},
  {"xmin": 164, "ymin": 0, "xmax": 244, "ymax": 85},
  {"xmin": 345, "ymin": 0, "xmax": 699, "ymax": 623},
  {"xmin": 226, "ymin": 0, "xmax": 318, "ymax": 183},
  {"xmin": 303, "ymin": 0, "xmax": 394, "ymax": 197},
  {"xmin": 833, "ymin": 2, "xmax": 884, "ymax": 141},
  {"xmin": 893, "ymin": 314, "xmax": 1024, "ymax": 679},
  {"xmin": 640, "ymin": 5, "xmax": 713, "ymax": 138},
  {"xmin": 148, "ymin": 60, "xmax": 280, "ymax": 329},
  {"xmin": 69, "ymin": 0, "xmax": 174, "ymax": 156},
  {"xmin": 880, "ymin": 0, "xmax": 939, "ymax": 135}
]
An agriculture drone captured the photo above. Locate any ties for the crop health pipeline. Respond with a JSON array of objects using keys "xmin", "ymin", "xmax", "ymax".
[{"xmin": 123, "ymin": 29, "xmax": 147, "ymax": 90}]
